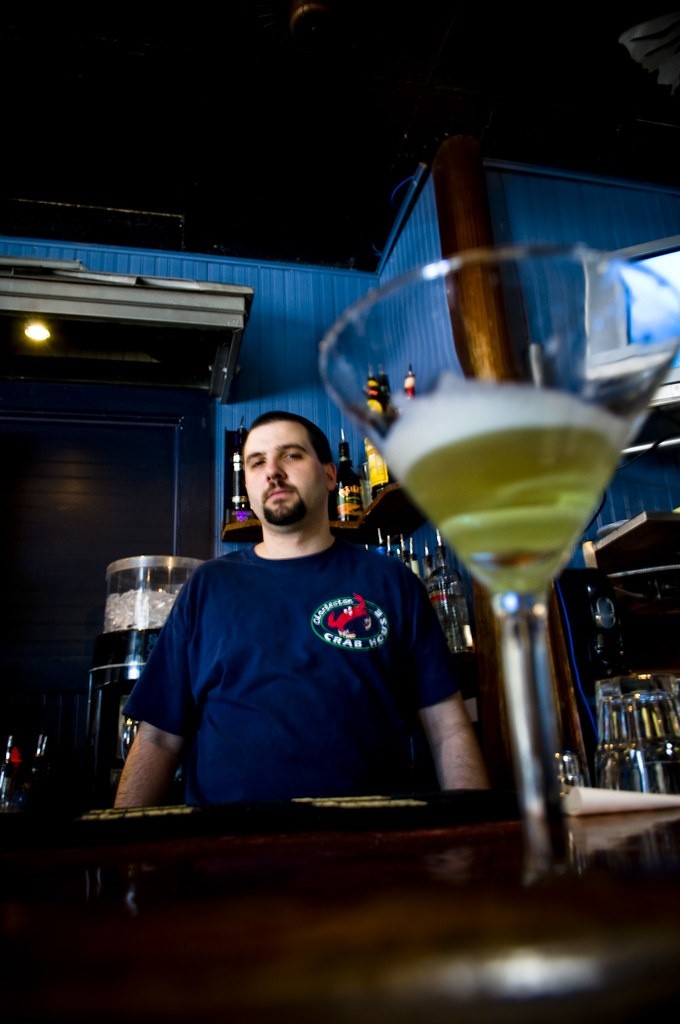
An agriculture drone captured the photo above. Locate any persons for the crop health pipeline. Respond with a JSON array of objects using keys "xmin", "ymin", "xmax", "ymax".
[{"xmin": 113, "ymin": 411, "xmax": 488, "ymax": 811}]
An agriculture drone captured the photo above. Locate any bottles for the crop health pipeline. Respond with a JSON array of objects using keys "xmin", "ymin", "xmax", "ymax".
[
  {"xmin": 337, "ymin": 441, "xmax": 363, "ymax": 522},
  {"xmin": 364, "ymin": 377, "xmax": 392, "ymax": 498},
  {"xmin": 377, "ymin": 374, "xmax": 391, "ymax": 408},
  {"xmin": 375, "ymin": 528, "xmax": 475, "ymax": 654},
  {"xmin": 230, "ymin": 429, "xmax": 249, "ymax": 510},
  {"xmin": 404, "ymin": 374, "xmax": 415, "ymax": 400}
]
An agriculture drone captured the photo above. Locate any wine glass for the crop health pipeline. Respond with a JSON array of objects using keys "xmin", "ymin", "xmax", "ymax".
[{"xmin": 318, "ymin": 243, "xmax": 680, "ymax": 890}]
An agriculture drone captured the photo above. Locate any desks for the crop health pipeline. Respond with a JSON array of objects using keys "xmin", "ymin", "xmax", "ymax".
[{"xmin": 0, "ymin": 789, "xmax": 680, "ymax": 1024}]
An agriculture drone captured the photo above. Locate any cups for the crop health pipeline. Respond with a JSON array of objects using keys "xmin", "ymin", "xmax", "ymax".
[
  {"xmin": 557, "ymin": 752, "xmax": 584, "ymax": 796},
  {"xmin": 594, "ymin": 672, "xmax": 680, "ymax": 798},
  {"xmin": 0, "ymin": 735, "xmax": 47, "ymax": 814}
]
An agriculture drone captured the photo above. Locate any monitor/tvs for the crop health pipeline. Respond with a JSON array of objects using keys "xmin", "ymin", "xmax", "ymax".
[{"xmin": 584, "ymin": 234, "xmax": 680, "ymax": 401}]
